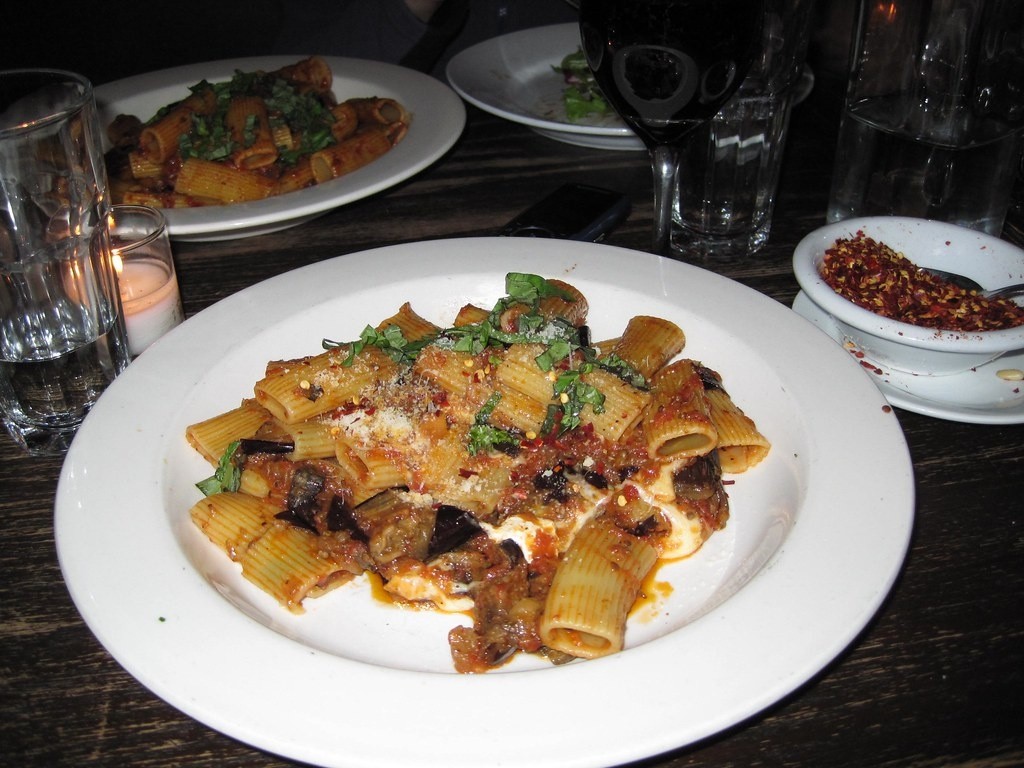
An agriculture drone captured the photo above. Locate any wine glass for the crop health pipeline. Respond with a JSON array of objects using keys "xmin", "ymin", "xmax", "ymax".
[{"xmin": 577, "ymin": 0, "xmax": 761, "ymax": 271}]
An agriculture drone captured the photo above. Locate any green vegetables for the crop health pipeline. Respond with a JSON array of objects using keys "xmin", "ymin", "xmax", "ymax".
[
  {"xmin": 194, "ymin": 271, "xmax": 652, "ymax": 500},
  {"xmin": 549, "ymin": 43, "xmax": 619, "ymax": 119},
  {"xmin": 140, "ymin": 65, "xmax": 339, "ymax": 174}
]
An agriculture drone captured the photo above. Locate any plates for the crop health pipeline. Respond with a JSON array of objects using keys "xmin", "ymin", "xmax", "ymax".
[
  {"xmin": 790, "ymin": 288, "xmax": 1024, "ymax": 425},
  {"xmin": 55, "ymin": 235, "xmax": 914, "ymax": 768},
  {"xmin": 447, "ymin": 23, "xmax": 813, "ymax": 150},
  {"xmin": 30, "ymin": 55, "xmax": 467, "ymax": 242}
]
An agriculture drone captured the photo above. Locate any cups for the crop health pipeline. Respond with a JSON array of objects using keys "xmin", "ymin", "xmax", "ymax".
[
  {"xmin": 666, "ymin": 0, "xmax": 816, "ymax": 254},
  {"xmin": 827, "ymin": 0, "xmax": 1024, "ymax": 237},
  {"xmin": 0, "ymin": 70, "xmax": 131, "ymax": 451}
]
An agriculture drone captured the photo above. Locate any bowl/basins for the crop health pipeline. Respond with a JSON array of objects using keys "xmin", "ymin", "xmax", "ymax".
[{"xmin": 791, "ymin": 215, "xmax": 1024, "ymax": 372}]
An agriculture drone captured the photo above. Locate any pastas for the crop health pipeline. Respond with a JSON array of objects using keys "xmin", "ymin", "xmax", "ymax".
[
  {"xmin": 184, "ymin": 278, "xmax": 771, "ymax": 658},
  {"xmin": 98, "ymin": 54, "xmax": 411, "ymax": 212}
]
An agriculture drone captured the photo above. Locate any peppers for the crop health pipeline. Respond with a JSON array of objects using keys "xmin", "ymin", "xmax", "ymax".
[{"xmin": 821, "ymin": 229, "xmax": 1024, "ymax": 333}]
